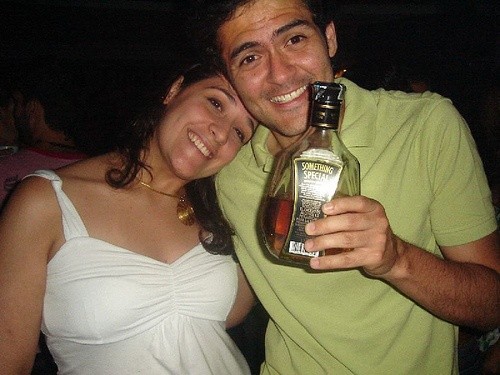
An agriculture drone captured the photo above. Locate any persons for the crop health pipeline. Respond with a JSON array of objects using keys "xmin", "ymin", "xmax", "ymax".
[
  {"xmin": 201, "ymin": 0, "xmax": 500, "ymax": 375},
  {"xmin": 0, "ymin": 75, "xmax": 99, "ymax": 206},
  {"xmin": 0, "ymin": 50, "xmax": 262, "ymax": 375}
]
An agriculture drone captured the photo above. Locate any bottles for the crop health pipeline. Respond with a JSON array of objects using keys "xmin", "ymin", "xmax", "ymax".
[{"xmin": 260, "ymin": 82, "xmax": 360, "ymax": 267}]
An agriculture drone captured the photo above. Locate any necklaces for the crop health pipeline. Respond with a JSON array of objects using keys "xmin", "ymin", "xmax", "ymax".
[{"xmin": 130, "ymin": 171, "xmax": 199, "ymax": 225}]
